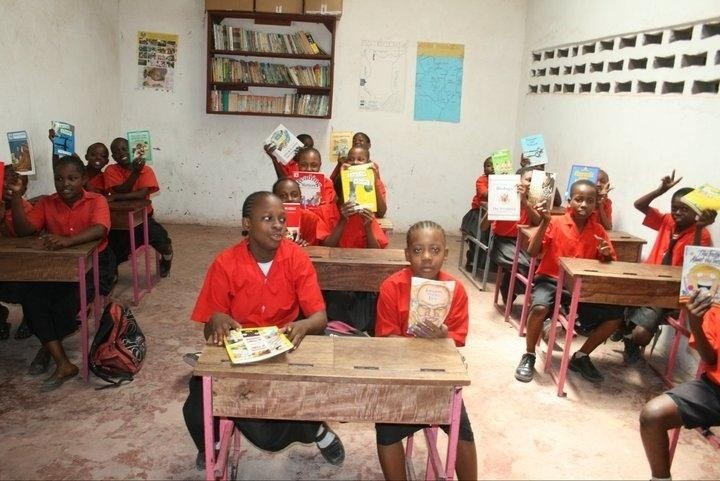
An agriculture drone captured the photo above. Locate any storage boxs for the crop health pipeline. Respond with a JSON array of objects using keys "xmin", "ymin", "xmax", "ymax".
[{"xmin": 204, "ymin": 0, "xmax": 344, "ymax": 20}]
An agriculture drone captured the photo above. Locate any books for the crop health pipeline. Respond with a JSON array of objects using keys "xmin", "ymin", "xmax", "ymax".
[
  {"xmin": 679, "ymin": 183, "xmax": 719, "ymax": 215},
  {"xmin": 210, "ymin": 22, "xmax": 330, "ymax": 119},
  {"xmin": 6, "ymin": 130, "xmax": 35, "ymax": 175},
  {"xmin": 521, "ymin": 134, "xmax": 548, "ymax": 168},
  {"xmin": 679, "ymin": 245, "xmax": 720, "ymax": 306},
  {"xmin": 51, "ymin": 122, "xmax": 76, "ymax": 158},
  {"xmin": 406, "ymin": 276, "xmax": 458, "ymax": 334},
  {"xmin": 266, "ymin": 123, "xmax": 306, "ymax": 167},
  {"xmin": 224, "ymin": 326, "xmax": 294, "ymax": 366},
  {"xmin": 127, "ymin": 131, "xmax": 151, "ymax": 161}
]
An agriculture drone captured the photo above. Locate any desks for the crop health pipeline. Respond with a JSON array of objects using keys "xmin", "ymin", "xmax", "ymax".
[
  {"xmin": 458, "ymin": 199, "xmax": 687, "ymax": 397},
  {"xmin": 192, "ymin": 217, "xmax": 474, "ymax": 476},
  {"xmin": 0, "ymin": 168, "xmax": 159, "ymax": 480}
]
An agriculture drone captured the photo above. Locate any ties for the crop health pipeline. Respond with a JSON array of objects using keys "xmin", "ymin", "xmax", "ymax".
[{"xmin": 662, "ymin": 229, "xmax": 683, "ymax": 266}]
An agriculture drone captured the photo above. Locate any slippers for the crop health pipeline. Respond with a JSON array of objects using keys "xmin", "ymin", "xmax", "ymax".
[
  {"xmin": 2, "ymin": 322, "xmax": 11, "ymax": 338},
  {"xmin": 14, "ymin": 321, "xmax": 33, "ymax": 339}
]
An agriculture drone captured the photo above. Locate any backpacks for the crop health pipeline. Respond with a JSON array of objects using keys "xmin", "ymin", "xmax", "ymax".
[{"xmin": 89, "ymin": 302, "xmax": 146, "ymax": 387}]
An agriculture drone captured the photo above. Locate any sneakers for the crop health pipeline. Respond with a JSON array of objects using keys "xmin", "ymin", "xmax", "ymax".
[
  {"xmin": 159, "ymin": 253, "xmax": 172, "ymax": 278},
  {"xmin": 30, "ymin": 347, "xmax": 51, "ymax": 376},
  {"xmin": 611, "ymin": 325, "xmax": 639, "ymax": 364},
  {"xmin": 40, "ymin": 365, "xmax": 78, "ymax": 392}
]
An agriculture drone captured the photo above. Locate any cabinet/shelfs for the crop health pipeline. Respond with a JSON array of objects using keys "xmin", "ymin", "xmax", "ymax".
[{"xmin": 204, "ymin": 11, "xmax": 337, "ymax": 119}]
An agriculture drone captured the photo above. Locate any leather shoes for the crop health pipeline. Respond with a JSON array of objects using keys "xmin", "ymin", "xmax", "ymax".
[
  {"xmin": 197, "ymin": 449, "xmax": 219, "ymax": 469},
  {"xmin": 516, "ymin": 353, "xmax": 535, "ymax": 382},
  {"xmin": 316, "ymin": 422, "xmax": 345, "ymax": 464},
  {"xmin": 568, "ymin": 353, "xmax": 604, "ymax": 382}
]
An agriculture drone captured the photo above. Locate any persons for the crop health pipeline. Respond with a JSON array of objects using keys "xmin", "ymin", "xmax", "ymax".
[
  {"xmin": 264, "ymin": 131, "xmax": 388, "ymax": 336},
  {"xmin": 372, "ymin": 219, "xmax": 479, "ymax": 481},
  {"xmin": 638, "ymin": 286, "xmax": 719, "ymax": 481},
  {"xmin": 182, "ymin": 190, "xmax": 346, "ymax": 473},
  {"xmin": 0, "ymin": 138, "xmax": 174, "ymax": 392},
  {"xmin": 463, "ymin": 152, "xmax": 621, "ymax": 383},
  {"xmin": 144, "ymin": 68, "xmax": 165, "ymax": 88},
  {"xmin": 612, "ymin": 170, "xmax": 718, "ymax": 363}
]
